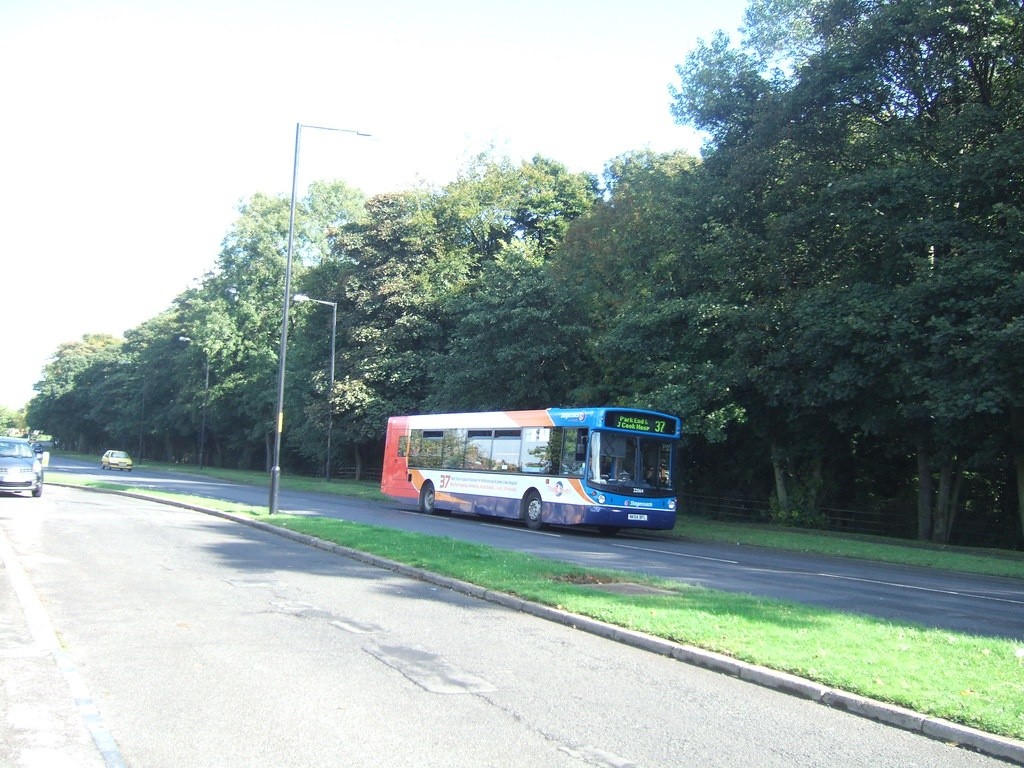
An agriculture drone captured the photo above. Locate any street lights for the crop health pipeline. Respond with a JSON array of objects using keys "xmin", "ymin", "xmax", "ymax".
[
  {"xmin": 179, "ymin": 336, "xmax": 209, "ymax": 470},
  {"xmin": 266, "ymin": 121, "xmax": 374, "ymax": 513},
  {"xmin": 291, "ymin": 294, "xmax": 337, "ymax": 482}
]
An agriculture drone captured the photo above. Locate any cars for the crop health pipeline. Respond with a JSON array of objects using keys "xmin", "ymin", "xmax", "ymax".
[
  {"xmin": 100, "ymin": 450, "xmax": 132, "ymax": 471},
  {"xmin": 31, "ymin": 443, "xmax": 44, "ymax": 455},
  {"xmin": 0, "ymin": 437, "xmax": 43, "ymax": 497}
]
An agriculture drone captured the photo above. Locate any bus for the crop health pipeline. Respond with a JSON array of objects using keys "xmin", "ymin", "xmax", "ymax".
[{"xmin": 380, "ymin": 407, "xmax": 681, "ymax": 537}]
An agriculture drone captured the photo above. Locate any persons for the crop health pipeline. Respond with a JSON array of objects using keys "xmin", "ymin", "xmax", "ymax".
[
  {"xmin": 398, "ymin": 437, "xmax": 513, "ymax": 471},
  {"xmin": 579, "ymin": 455, "xmax": 593, "ymax": 479},
  {"xmin": 28, "ymin": 438, "xmax": 36, "ymax": 457}
]
